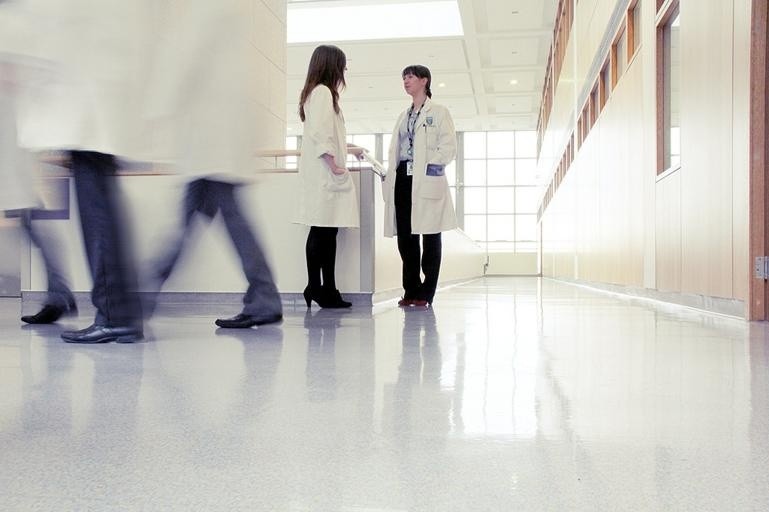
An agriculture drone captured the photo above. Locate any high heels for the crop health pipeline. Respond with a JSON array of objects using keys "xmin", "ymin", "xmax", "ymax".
[
  {"xmin": 396, "ymin": 298, "xmax": 433, "ymax": 308},
  {"xmin": 304, "ymin": 286, "xmax": 353, "ymax": 311}
]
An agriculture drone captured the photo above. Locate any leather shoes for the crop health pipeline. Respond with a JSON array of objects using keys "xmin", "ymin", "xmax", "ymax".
[
  {"xmin": 58, "ymin": 323, "xmax": 146, "ymax": 344},
  {"xmin": 214, "ymin": 311, "xmax": 285, "ymax": 327},
  {"xmin": 18, "ymin": 300, "xmax": 78, "ymax": 323}
]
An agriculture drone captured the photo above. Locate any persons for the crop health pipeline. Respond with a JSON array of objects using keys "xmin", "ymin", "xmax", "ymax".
[
  {"xmin": 18, "ymin": 117, "xmax": 284, "ymax": 345},
  {"xmin": 292, "ymin": 45, "xmax": 370, "ymax": 310},
  {"xmin": 380, "ymin": 66, "xmax": 458, "ymax": 307}
]
